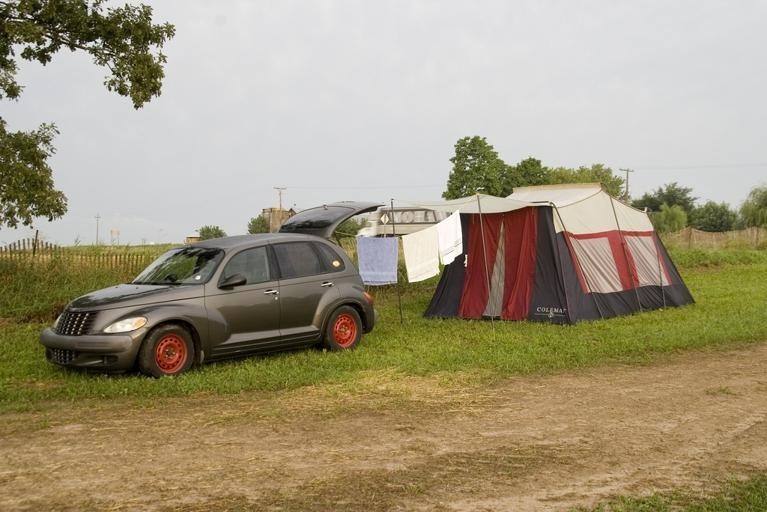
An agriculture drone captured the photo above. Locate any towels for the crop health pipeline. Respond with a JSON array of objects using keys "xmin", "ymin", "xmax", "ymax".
[
  {"xmin": 400, "ymin": 224, "xmax": 440, "ymax": 283},
  {"xmin": 356, "ymin": 235, "xmax": 399, "ymax": 286},
  {"xmin": 437, "ymin": 210, "xmax": 464, "ymax": 266}
]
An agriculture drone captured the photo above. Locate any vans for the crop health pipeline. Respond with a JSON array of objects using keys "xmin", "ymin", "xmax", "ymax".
[{"xmin": 356, "ymin": 206, "xmax": 440, "ymax": 237}]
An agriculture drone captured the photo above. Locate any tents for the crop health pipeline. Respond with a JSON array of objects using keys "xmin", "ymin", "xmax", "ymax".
[{"xmin": 422, "ymin": 187, "xmax": 694, "ymax": 326}]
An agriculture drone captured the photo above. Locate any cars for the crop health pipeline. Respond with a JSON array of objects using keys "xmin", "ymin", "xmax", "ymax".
[{"xmin": 38, "ymin": 199, "xmax": 384, "ymax": 379}]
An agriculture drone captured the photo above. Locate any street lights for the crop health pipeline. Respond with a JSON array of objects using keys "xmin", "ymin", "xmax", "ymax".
[
  {"xmin": 619, "ymin": 168, "xmax": 634, "ymax": 203},
  {"xmin": 274, "ymin": 186, "xmax": 286, "ymax": 225},
  {"xmin": 93, "ymin": 212, "xmax": 101, "ymax": 246}
]
